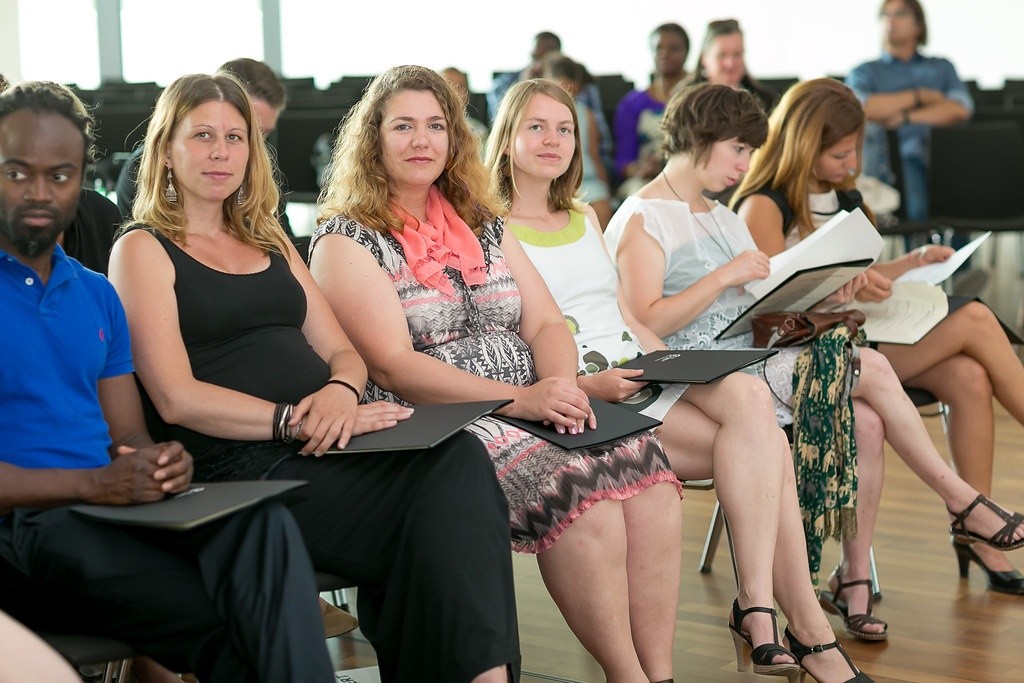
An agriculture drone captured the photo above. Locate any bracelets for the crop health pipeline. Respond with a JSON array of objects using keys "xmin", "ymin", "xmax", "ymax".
[
  {"xmin": 913, "ymin": 88, "xmax": 922, "ymax": 107},
  {"xmin": 324, "ymin": 380, "xmax": 359, "ymax": 402},
  {"xmin": 903, "ymin": 110, "xmax": 909, "ymax": 123},
  {"xmin": 273, "ymin": 403, "xmax": 301, "ymax": 443}
]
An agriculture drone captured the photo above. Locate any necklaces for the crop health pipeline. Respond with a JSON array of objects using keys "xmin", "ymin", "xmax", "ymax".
[{"xmin": 663, "ymin": 171, "xmax": 734, "ymax": 260}]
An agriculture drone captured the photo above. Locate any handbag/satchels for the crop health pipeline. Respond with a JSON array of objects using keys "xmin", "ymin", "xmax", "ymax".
[{"xmin": 751, "ymin": 308, "xmax": 866, "ymax": 348}]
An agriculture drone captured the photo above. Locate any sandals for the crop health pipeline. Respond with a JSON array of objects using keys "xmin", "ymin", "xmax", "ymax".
[
  {"xmin": 821, "ymin": 564, "xmax": 888, "ymax": 640},
  {"xmin": 947, "ymin": 493, "xmax": 1024, "ymax": 551}
]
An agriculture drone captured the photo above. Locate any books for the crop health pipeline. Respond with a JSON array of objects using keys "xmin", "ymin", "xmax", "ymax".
[
  {"xmin": 487, "ymin": 396, "xmax": 663, "ymax": 452},
  {"xmin": 832, "ymin": 231, "xmax": 1024, "ymax": 349},
  {"xmin": 713, "ymin": 208, "xmax": 885, "ymax": 340},
  {"xmin": 69, "ymin": 481, "xmax": 308, "ymax": 530},
  {"xmin": 313, "ymin": 399, "xmax": 513, "ymax": 454},
  {"xmin": 615, "ymin": 350, "xmax": 779, "ymax": 385}
]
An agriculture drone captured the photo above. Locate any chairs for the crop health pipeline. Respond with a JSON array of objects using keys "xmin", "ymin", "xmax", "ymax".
[{"xmin": 48, "ymin": 75, "xmax": 1023, "ymax": 683}]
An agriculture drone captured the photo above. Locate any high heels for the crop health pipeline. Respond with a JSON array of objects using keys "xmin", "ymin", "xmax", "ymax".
[
  {"xmin": 728, "ymin": 598, "xmax": 801, "ymax": 675},
  {"xmin": 783, "ymin": 624, "xmax": 874, "ymax": 683},
  {"xmin": 953, "ymin": 539, "xmax": 1024, "ymax": 595}
]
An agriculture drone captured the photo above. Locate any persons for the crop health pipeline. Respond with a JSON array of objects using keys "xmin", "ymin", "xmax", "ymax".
[
  {"xmin": 487, "ymin": 32, "xmax": 613, "ymax": 231},
  {"xmin": 729, "ymin": 78, "xmax": 1024, "ymax": 598},
  {"xmin": 487, "ymin": 78, "xmax": 874, "ymax": 683},
  {"xmin": 602, "ymin": 83, "xmax": 1023, "ymax": 641},
  {"xmin": 701, "ymin": 20, "xmax": 772, "ymax": 110},
  {"xmin": 1, "ymin": 82, "xmax": 335, "ymax": 683},
  {"xmin": 107, "ymin": 74, "xmax": 522, "ymax": 683},
  {"xmin": 311, "ymin": 66, "xmax": 682, "ymax": 683},
  {"xmin": 117, "ymin": 58, "xmax": 285, "ymax": 223},
  {"xmin": 846, "ymin": 0, "xmax": 977, "ymax": 220},
  {"xmin": 60, "ymin": 190, "xmax": 121, "ymax": 278},
  {"xmin": 613, "ymin": 23, "xmax": 688, "ymax": 190},
  {"xmin": 441, "ymin": 69, "xmax": 488, "ymax": 141}
]
